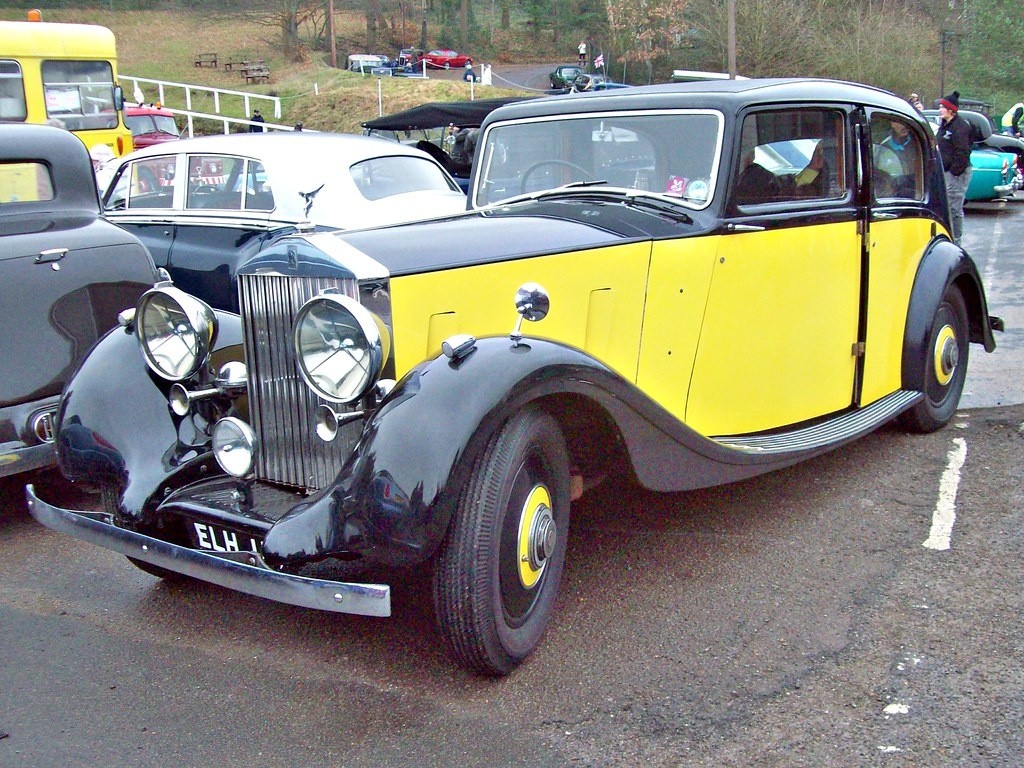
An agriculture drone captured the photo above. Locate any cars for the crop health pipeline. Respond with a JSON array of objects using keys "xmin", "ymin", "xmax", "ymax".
[
  {"xmin": 1, "ymin": 76, "xmax": 1024, "ymax": 678},
  {"xmin": 549, "ymin": 66, "xmax": 585, "ymax": 92},
  {"xmin": 345, "ymin": 47, "xmax": 474, "ymax": 75}
]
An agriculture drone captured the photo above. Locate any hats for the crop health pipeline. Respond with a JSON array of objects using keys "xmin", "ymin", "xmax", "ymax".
[
  {"xmin": 937, "ymin": 91, "xmax": 960, "ymax": 112},
  {"xmin": 910, "ymin": 93, "xmax": 918, "ymax": 99}
]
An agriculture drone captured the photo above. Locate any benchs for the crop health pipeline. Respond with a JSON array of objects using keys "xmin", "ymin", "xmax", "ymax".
[
  {"xmin": 223, "ymin": 56, "xmax": 270, "ymax": 84},
  {"xmin": 194, "ymin": 53, "xmax": 218, "ymax": 68}
]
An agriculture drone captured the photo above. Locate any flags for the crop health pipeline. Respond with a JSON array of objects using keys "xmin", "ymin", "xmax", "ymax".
[{"xmin": 594, "ymin": 54, "xmax": 604, "ymax": 68}]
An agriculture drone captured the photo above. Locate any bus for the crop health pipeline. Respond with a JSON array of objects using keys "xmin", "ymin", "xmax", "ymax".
[
  {"xmin": 97, "ymin": 107, "xmax": 230, "ymax": 196},
  {"xmin": 0, "ymin": 20, "xmax": 140, "ymax": 203}
]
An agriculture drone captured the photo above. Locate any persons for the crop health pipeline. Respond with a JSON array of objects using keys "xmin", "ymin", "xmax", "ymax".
[
  {"xmin": 464, "ymin": 65, "xmax": 476, "ymax": 82},
  {"xmin": 796, "ymin": 117, "xmax": 916, "ymax": 198},
  {"xmin": 578, "ymin": 40, "xmax": 587, "ymax": 65},
  {"xmin": 293, "ymin": 122, "xmax": 302, "ymax": 132},
  {"xmin": 1001, "ymin": 101, "xmax": 1024, "ymax": 139},
  {"xmin": 449, "ymin": 127, "xmax": 479, "ymax": 165},
  {"xmin": 909, "ymin": 94, "xmax": 925, "ymax": 111},
  {"xmin": 937, "ymin": 91, "xmax": 975, "ymax": 245},
  {"xmin": 249, "ymin": 109, "xmax": 265, "ymax": 133},
  {"xmin": 404, "ymin": 47, "xmax": 418, "ymax": 74}
]
